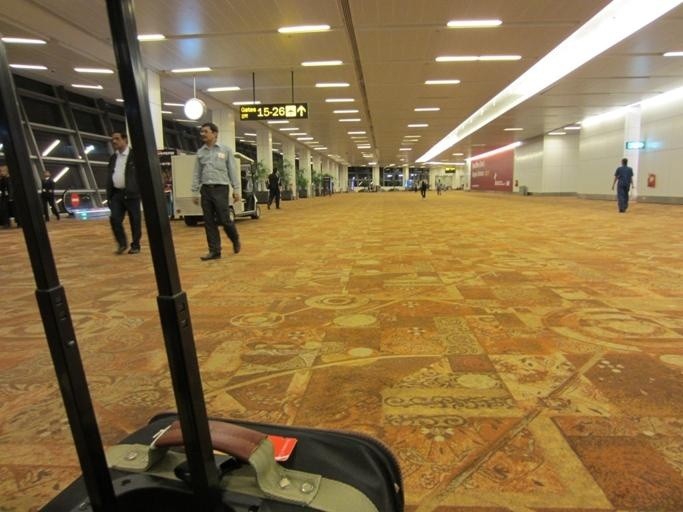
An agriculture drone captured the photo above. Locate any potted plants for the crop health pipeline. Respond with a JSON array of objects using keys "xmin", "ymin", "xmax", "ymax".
[{"xmin": 249, "ymin": 159, "xmax": 324, "ymax": 204}]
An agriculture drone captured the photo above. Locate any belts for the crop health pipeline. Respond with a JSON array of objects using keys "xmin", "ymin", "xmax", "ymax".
[{"xmin": 204, "ymin": 184, "xmax": 225, "ymax": 188}]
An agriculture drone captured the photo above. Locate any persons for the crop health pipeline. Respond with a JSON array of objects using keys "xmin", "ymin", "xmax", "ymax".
[
  {"xmin": 436, "ymin": 179, "xmax": 443, "ymax": 196},
  {"xmin": 104, "ymin": 131, "xmax": 143, "ymax": 255},
  {"xmin": 265, "ymin": 167, "xmax": 281, "ymax": 210},
  {"xmin": 418, "ymin": 175, "xmax": 428, "ymax": 198},
  {"xmin": 40, "ymin": 168, "xmax": 59, "ymax": 222},
  {"xmin": 0, "ymin": 161, "xmax": 22, "ymax": 229},
  {"xmin": 240, "ymin": 169, "xmax": 253, "ymax": 212},
  {"xmin": 611, "ymin": 157, "xmax": 636, "ymax": 213},
  {"xmin": 190, "ymin": 121, "xmax": 242, "ymax": 261}
]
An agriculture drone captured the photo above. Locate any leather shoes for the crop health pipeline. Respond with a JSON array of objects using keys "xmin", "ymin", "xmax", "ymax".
[
  {"xmin": 233, "ymin": 236, "xmax": 240, "ymax": 252},
  {"xmin": 201, "ymin": 252, "xmax": 220, "ymax": 260},
  {"xmin": 129, "ymin": 247, "xmax": 139, "ymax": 254}
]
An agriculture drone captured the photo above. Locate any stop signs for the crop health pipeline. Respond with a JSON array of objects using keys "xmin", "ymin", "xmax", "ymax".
[{"xmin": 70, "ymin": 193, "xmax": 80, "ymax": 208}]
